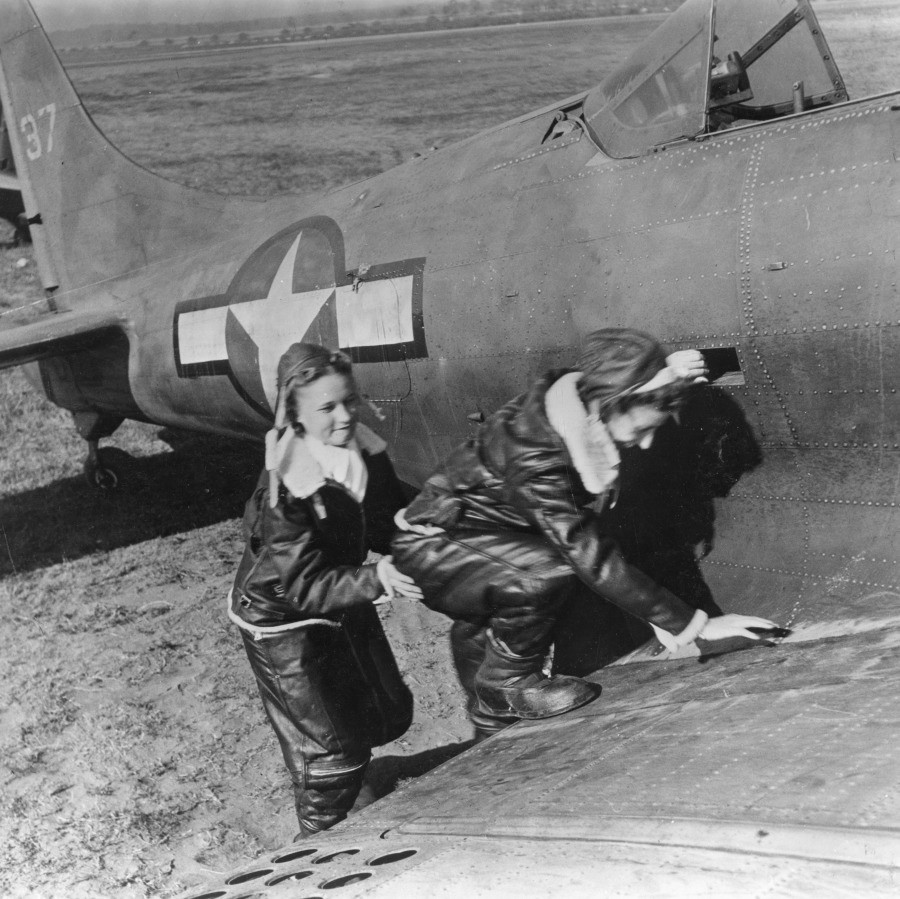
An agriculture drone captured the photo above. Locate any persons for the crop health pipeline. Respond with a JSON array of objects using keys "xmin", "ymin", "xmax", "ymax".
[
  {"xmin": 225, "ymin": 343, "xmax": 424, "ymax": 839},
  {"xmin": 391, "ymin": 328, "xmax": 781, "ymax": 742}
]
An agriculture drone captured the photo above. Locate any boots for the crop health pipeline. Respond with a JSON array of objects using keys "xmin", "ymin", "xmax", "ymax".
[{"xmin": 467, "ymin": 628, "xmax": 595, "ymax": 731}]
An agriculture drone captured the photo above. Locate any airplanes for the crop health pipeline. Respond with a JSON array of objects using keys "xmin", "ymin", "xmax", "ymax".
[{"xmin": 0, "ymin": 0, "xmax": 900, "ymax": 899}]
